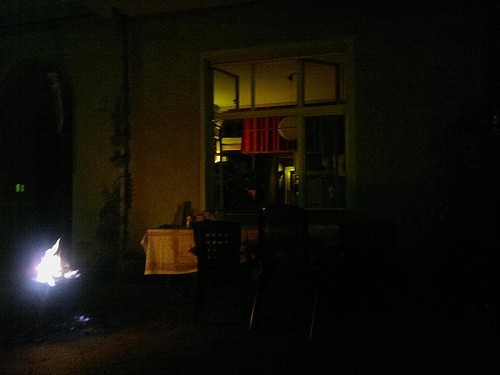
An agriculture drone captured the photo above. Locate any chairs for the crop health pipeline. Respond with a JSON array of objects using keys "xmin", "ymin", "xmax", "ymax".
[
  {"xmin": 249, "ymin": 203, "xmax": 339, "ymax": 345},
  {"xmin": 190, "ymin": 220, "xmax": 257, "ymax": 326}
]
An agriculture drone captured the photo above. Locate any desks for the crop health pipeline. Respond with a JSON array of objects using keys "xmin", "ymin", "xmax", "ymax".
[{"xmin": 139, "ymin": 224, "xmax": 343, "ymax": 330}]
